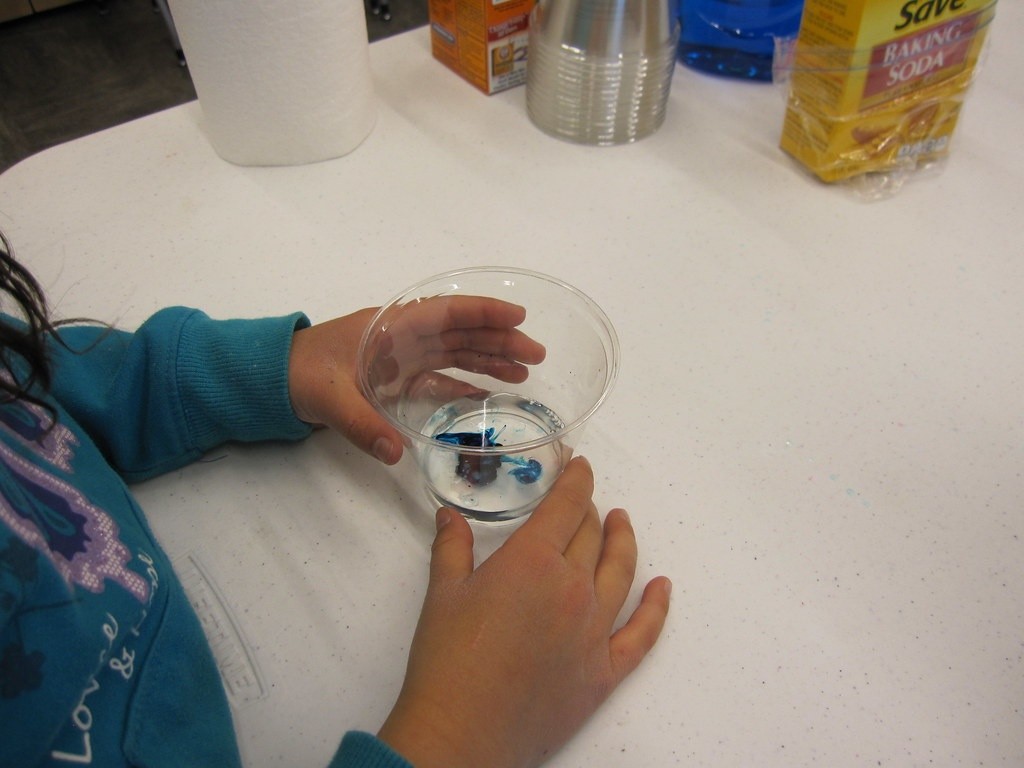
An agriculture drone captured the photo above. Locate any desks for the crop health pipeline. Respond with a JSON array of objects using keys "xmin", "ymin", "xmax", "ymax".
[{"xmin": 0, "ymin": 1, "xmax": 1022, "ymax": 768}]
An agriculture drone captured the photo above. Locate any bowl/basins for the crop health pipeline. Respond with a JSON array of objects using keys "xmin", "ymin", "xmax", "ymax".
[{"xmin": 358, "ymin": 265, "xmax": 622, "ymax": 524}]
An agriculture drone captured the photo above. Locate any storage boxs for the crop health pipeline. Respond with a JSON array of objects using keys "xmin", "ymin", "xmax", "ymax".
[
  {"xmin": 777, "ymin": 0, "xmax": 996, "ymax": 183},
  {"xmin": 428, "ymin": 0, "xmax": 536, "ymax": 97}
]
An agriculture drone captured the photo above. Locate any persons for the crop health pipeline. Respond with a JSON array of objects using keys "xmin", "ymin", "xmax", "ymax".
[{"xmin": 0, "ymin": 232, "xmax": 673, "ymax": 768}]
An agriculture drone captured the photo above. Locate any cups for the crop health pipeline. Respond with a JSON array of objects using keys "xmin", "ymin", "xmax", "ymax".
[{"xmin": 524, "ymin": 0, "xmax": 683, "ymax": 149}]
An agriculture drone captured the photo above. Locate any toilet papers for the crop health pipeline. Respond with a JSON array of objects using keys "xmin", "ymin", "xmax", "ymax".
[{"xmin": 165, "ymin": 0, "xmax": 380, "ymax": 169}]
